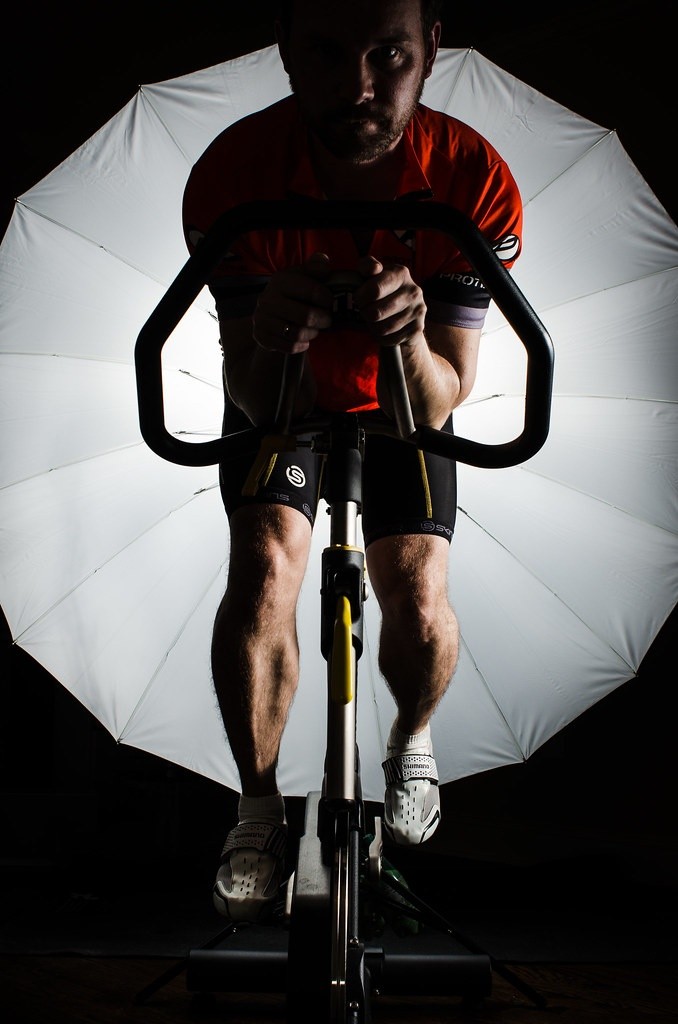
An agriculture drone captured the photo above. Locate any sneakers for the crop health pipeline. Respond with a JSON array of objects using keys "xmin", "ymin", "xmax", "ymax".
[
  {"xmin": 212, "ymin": 817, "xmax": 289, "ymax": 920},
  {"xmin": 381, "ymin": 742, "xmax": 442, "ymax": 847}
]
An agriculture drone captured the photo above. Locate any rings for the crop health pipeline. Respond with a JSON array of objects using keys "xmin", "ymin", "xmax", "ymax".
[{"xmin": 280, "ymin": 322, "xmax": 289, "ymax": 337}]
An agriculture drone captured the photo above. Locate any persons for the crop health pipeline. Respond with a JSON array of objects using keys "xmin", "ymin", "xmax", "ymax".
[{"xmin": 183, "ymin": 0, "xmax": 518, "ymax": 920}]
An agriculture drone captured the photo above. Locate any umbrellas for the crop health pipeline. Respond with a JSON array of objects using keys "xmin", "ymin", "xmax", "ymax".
[{"xmin": 3, "ymin": 41, "xmax": 677, "ymax": 805}]
